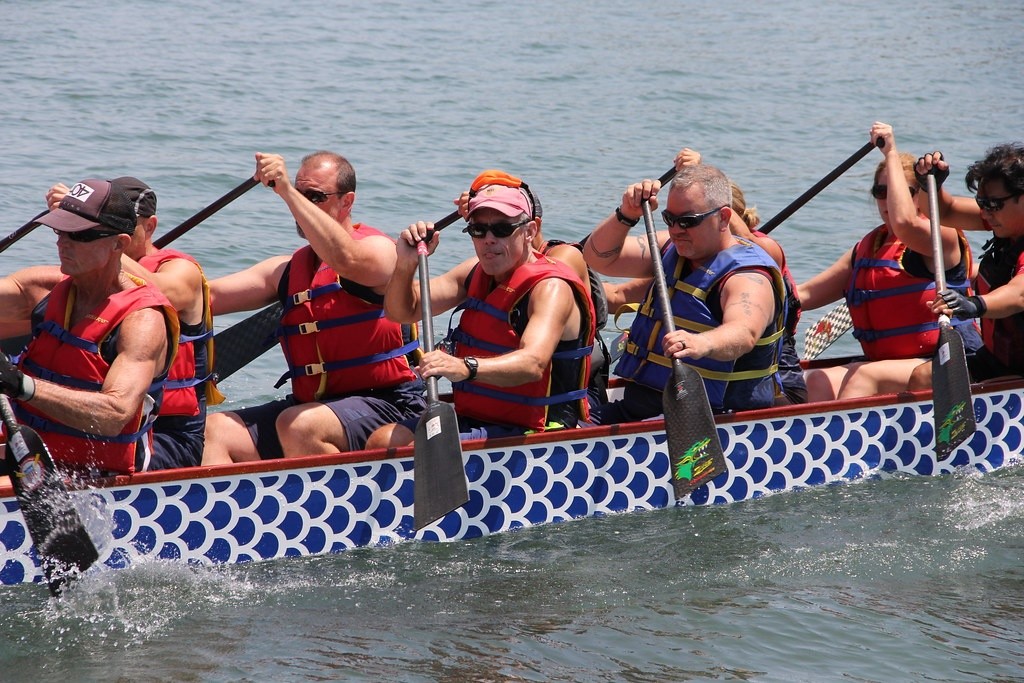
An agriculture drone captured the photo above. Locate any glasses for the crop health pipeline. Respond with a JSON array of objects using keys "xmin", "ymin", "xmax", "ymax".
[
  {"xmin": 976, "ymin": 193, "xmax": 1016, "ymax": 212},
  {"xmin": 661, "ymin": 204, "xmax": 731, "ymax": 229},
  {"xmin": 298, "ymin": 191, "xmax": 346, "ymax": 204},
  {"xmin": 871, "ymin": 184, "xmax": 919, "ymax": 198},
  {"xmin": 467, "ymin": 219, "xmax": 531, "ymax": 238},
  {"xmin": 53, "ymin": 228, "xmax": 133, "ymax": 243}
]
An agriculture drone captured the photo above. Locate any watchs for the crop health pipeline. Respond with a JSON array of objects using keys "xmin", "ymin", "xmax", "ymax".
[{"xmin": 463, "ymin": 356, "xmax": 478, "ymax": 383}]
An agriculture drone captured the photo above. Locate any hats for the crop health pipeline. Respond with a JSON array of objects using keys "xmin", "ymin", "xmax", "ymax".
[
  {"xmin": 521, "ymin": 183, "xmax": 542, "ymax": 217},
  {"xmin": 464, "ymin": 179, "xmax": 532, "ymax": 219},
  {"xmin": 113, "ymin": 177, "xmax": 157, "ymax": 218},
  {"xmin": 32, "ymin": 178, "xmax": 138, "ymax": 235}
]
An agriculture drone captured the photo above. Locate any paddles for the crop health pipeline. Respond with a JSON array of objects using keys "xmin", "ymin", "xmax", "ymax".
[
  {"xmin": 152, "ymin": 175, "xmax": 278, "ymax": 250},
  {"xmin": 0, "ymin": 392, "xmax": 102, "ymax": 601},
  {"xmin": 756, "ymin": 135, "xmax": 887, "ymax": 235},
  {"xmin": 920, "ymin": 161, "xmax": 977, "ymax": 462},
  {"xmin": 407, "ymin": 226, "xmax": 472, "ymax": 533},
  {"xmin": 579, "ymin": 168, "xmax": 680, "ymax": 246},
  {"xmin": 212, "ymin": 212, "xmax": 461, "ymax": 385},
  {"xmin": 0, "ymin": 208, "xmax": 51, "ymax": 255},
  {"xmin": 636, "ymin": 189, "xmax": 726, "ymax": 502}
]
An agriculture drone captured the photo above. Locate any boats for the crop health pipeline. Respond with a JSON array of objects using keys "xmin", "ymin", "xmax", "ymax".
[{"xmin": 0, "ymin": 354, "xmax": 1023, "ymax": 588}]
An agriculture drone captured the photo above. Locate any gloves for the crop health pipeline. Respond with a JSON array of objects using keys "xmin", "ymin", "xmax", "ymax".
[{"xmin": 0, "ymin": 351, "xmax": 35, "ymax": 402}]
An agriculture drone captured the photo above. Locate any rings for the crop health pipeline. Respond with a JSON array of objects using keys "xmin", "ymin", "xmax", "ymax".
[{"xmin": 679, "ymin": 341, "xmax": 686, "ymax": 350}]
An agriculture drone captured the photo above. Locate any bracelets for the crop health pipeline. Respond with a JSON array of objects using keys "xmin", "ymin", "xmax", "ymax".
[{"xmin": 616, "ymin": 206, "xmax": 640, "ymax": 227}]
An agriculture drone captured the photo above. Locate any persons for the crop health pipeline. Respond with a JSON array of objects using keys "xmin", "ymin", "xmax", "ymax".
[
  {"xmin": 0, "ymin": 179, "xmax": 180, "ymax": 486},
  {"xmin": 45, "ymin": 176, "xmax": 207, "ymax": 473},
  {"xmin": 582, "ymin": 148, "xmax": 807, "ymax": 425},
  {"xmin": 200, "ymin": 151, "xmax": 424, "ymax": 467},
  {"xmin": 907, "ymin": 140, "xmax": 1024, "ymax": 392},
  {"xmin": 276, "ymin": 170, "xmax": 608, "ymax": 458},
  {"xmin": 796, "ymin": 122, "xmax": 983, "ymax": 404}
]
